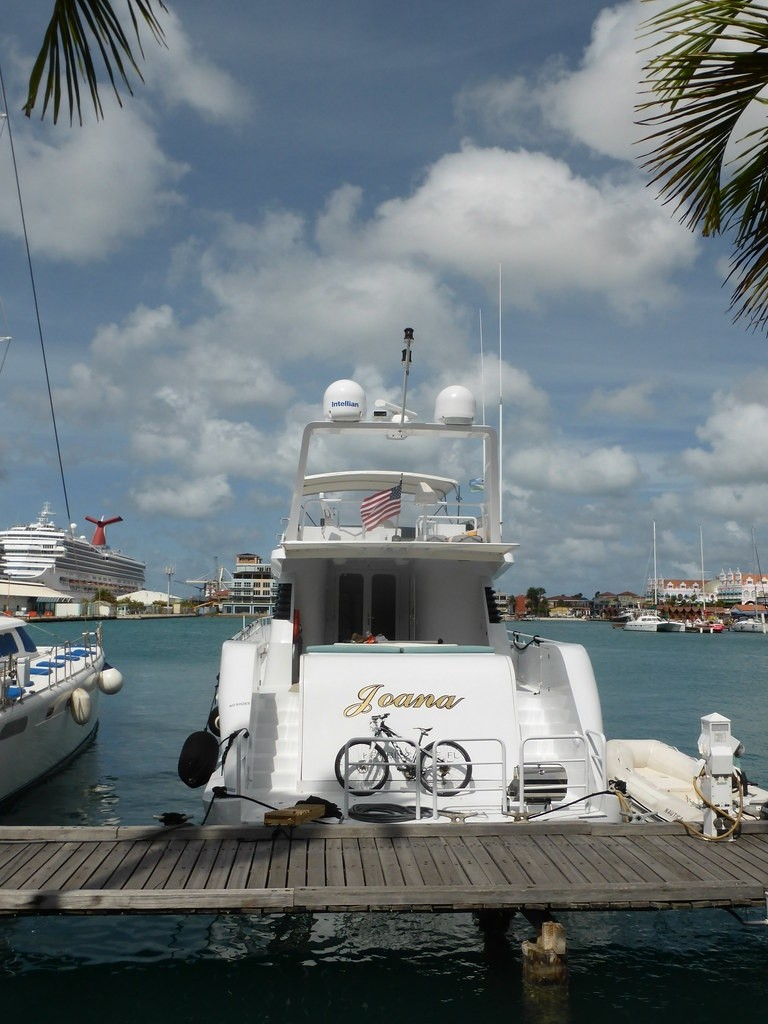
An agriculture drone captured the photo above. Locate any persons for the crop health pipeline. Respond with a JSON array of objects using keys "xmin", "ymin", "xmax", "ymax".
[
  {"xmin": 363, "ymin": 631, "xmax": 375, "ymax": 644},
  {"xmin": 687, "ymin": 615, "xmax": 705, "ymax": 622},
  {"xmin": 452, "ymin": 523, "xmax": 483, "ymax": 543},
  {"xmin": 350, "ymin": 633, "xmax": 364, "ymax": 643}
]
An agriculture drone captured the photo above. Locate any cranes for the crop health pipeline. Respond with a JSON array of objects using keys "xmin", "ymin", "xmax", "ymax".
[{"xmin": 184, "ymin": 554, "xmax": 233, "ymax": 587}]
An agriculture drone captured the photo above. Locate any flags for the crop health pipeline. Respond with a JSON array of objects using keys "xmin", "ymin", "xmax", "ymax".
[{"xmin": 360, "ymin": 485, "xmax": 402, "ymax": 531}]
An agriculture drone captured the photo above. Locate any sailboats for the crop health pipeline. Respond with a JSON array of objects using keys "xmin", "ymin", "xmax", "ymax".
[{"xmin": 622, "ymin": 522, "xmax": 768, "ymax": 633}]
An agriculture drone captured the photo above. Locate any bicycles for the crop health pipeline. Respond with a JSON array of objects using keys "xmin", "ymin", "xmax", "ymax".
[{"xmin": 334, "ymin": 713, "xmax": 473, "ymax": 798}]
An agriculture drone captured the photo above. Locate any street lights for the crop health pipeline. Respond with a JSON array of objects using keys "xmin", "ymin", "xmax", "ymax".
[{"xmin": 164, "ymin": 563, "xmax": 176, "ymax": 609}]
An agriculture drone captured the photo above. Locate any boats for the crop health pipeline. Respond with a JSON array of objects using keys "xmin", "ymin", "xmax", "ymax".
[
  {"xmin": 0, "ymin": 616, "xmax": 122, "ymax": 813},
  {"xmin": 175, "ymin": 322, "xmax": 631, "ymax": 824},
  {"xmin": 603, "ymin": 726, "xmax": 767, "ymax": 822}
]
